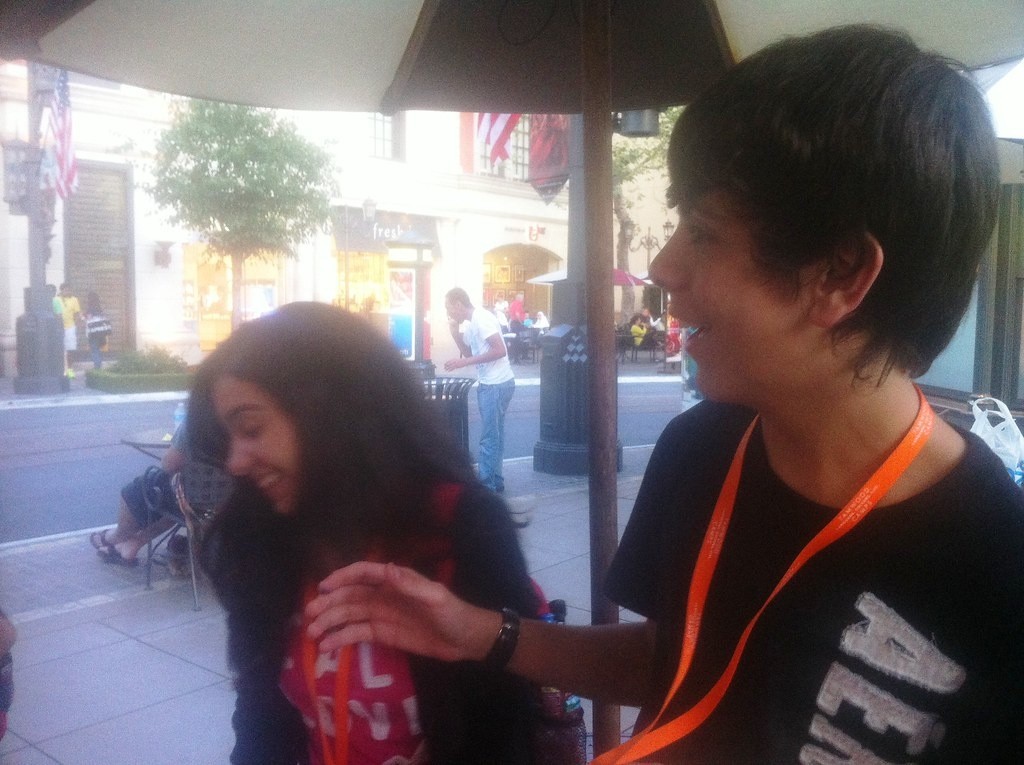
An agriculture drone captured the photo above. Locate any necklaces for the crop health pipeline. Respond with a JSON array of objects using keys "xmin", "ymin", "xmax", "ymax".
[{"xmin": 301, "ymin": 541, "xmax": 384, "ymax": 765}]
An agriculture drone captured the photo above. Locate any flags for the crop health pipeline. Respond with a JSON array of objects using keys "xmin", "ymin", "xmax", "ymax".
[
  {"xmin": 477, "ymin": 113, "xmax": 522, "ymax": 168},
  {"xmin": 40, "ymin": 70, "xmax": 79, "ymax": 204}
]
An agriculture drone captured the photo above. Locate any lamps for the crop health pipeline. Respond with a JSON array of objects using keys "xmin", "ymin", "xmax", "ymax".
[{"xmin": 157, "ymin": 241, "xmax": 174, "ymax": 270}]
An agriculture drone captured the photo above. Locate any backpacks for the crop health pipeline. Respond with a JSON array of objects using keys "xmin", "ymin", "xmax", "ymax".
[{"xmin": 409, "ymin": 475, "xmax": 587, "ymax": 765}]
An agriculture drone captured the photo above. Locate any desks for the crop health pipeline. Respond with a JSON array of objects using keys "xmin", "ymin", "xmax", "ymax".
[{"xmin": 120, "ymin": 428, "xmax": 175, "ymax": 461}]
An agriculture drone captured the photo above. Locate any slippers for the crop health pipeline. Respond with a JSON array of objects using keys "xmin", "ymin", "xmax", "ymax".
[
  {"xmin": 97, "ymin": 545, "xmax": 139, "ymax": 566},
  {"xmin": 89, "ymin": 528, "xmax": 114, "ymax": 550}
]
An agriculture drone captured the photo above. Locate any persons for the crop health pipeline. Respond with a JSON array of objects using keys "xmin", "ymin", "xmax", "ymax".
[
  {"xmin": 0, "ymin": 611, "xmax": 17, "ymax": 740},
  {"xmin": 91, "ymin": 416, "xmax": 228, "ymax": 566},
  {"xmin": 185, "ymin": 301, "xmax": 545, "ymax": 765},
  {"xmin": 444, "ymin": 289, "xmax": 516, "ymax": 494},
  {"xmin": 305, "ymin": 22, "xmax": 1024, "ymax": 765},
  {"xmin": 494, "ymin": 292, "xmax": 550, "ymax": 359},
  {"xmin": 45, "ymin": 283, "xmax": 111, "ymax": 378},
  {"xmin": 629, "ymin": 308, "xmax": 666, "ymax": 351}
]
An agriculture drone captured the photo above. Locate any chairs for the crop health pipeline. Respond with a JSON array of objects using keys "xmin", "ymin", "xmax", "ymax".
[
  {"xmin": 505, "ymin": 327, "xmax": 544, "ymax": 364},
  {"xmin": 617, "ymin": 325, "xmax": 665, "ymax": 362},
  {"xmin": 140, "ymin": 465, "xmax": 203, "ymax": 612}
]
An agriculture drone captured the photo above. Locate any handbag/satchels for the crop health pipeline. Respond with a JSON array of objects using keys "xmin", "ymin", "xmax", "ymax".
[
  {"xmin": 967, "ymin": 397, "xmax": 1024, "ymax": 489},
  {"xmin": 100, "ymin": 334, "xmax": 110, "ymax": 352}
]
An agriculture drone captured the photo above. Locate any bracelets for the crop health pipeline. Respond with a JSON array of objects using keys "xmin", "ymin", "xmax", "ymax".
[{"xmin": 474, "ymin": 606, "xmax": 522, "ymax": 671}]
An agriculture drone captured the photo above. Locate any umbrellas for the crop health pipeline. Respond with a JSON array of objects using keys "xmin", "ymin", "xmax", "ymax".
[
  {"xmin": 639, "ymin": 275, "xmax": 667, "ymax": 314},
  {"xmin": 0, "ymin": 0, "xmax": 1024, "ymax": 765},
  {"xmin": 526, "ymin": 265, "xmax": 650, "ymax": 287}
]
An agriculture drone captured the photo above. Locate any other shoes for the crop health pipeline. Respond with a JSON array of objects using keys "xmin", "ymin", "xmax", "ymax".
[
  {"xmin": 496, "ymin": 484, "xmax": 505, "ymax": 492},
  {"xmin": 64, "ymin": 368, "xmax": 75, "ymax": 379}
]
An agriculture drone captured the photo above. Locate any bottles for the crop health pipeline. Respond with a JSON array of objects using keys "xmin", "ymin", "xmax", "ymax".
[{"xmin": 538, "ymin": 599, "xmax": 581, "ymax": 714}]
